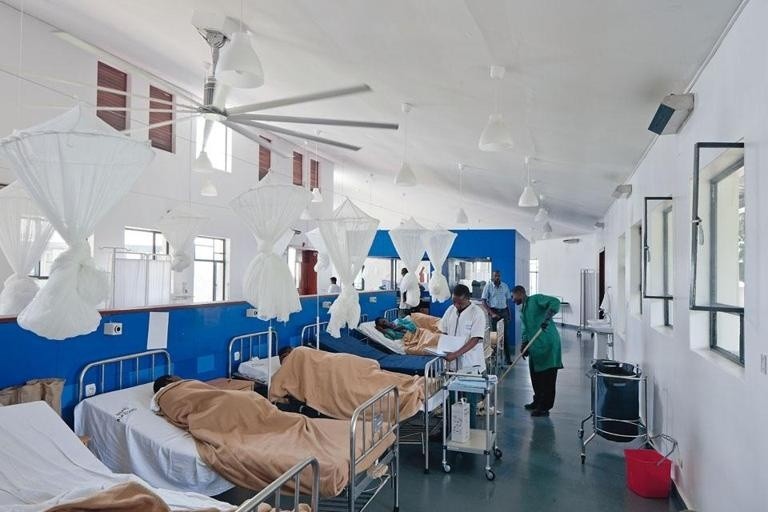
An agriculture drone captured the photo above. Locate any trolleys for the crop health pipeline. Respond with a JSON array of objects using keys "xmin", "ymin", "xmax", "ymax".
[{"xmin": 435, "ymin": 365, "xmax": 503, "ymax": 482}]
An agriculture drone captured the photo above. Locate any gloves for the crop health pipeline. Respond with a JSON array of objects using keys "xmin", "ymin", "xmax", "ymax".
[
  {"xmin": 541, "ymin": 319, "xmax": 548, "ymax": 331},
  {"xmin": 520, "ymin": 343, "xmax": 529, "ymax": 360}
]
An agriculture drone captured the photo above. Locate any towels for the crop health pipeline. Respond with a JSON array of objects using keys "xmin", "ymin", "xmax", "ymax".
[{"xmin": 600, "ymin": 287, "xmax": 610, "ymax": 314}]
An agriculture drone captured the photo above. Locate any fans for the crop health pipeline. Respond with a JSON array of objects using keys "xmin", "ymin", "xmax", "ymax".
[{"xmin": 25, "ymin": 32, "xmax": 399, "ymax": 157}]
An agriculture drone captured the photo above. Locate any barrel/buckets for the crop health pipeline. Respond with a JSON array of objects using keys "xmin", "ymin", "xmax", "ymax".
[{"xmin": 622, "ymin": 432, "xmax": 678, "ymax": 498}]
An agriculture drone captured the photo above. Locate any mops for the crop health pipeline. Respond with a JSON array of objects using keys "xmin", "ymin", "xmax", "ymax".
[{"xmin": 475, "ymin": 321, "xmax": 551, "ymax": 415}]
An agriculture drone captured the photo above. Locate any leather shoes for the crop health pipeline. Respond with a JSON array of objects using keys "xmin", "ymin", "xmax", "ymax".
[
  {"xmin": 524, "ymin": 400, "xmax": 550, "ymax": 417},
  {"xmin": 504, "ymin": 356, "xmax": 513, "ymax": 365}
]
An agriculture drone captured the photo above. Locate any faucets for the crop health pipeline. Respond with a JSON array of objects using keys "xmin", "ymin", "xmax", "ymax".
[
  {"xmin": 602, "ymin": 311, "xmax": 611, "ymax": 324},
  {"xmin": 556, "ymin": 296, "xmax": 563, "ymax": 302},
  {"xmin": 598, "ymin": 309, "xmax": 605, "ymax": 313}
]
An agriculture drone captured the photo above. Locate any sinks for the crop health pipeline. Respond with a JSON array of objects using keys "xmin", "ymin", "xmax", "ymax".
[
  {"xmin": 560, "ymin": 302, "xmax": 569, "ymax": 307},
  {"xmin": 586, "ymin": 318, "xmax": 606, "ymax": 324},
  {"xmin": 588, "ymin": 324, "xmax": 614, "ymax": 333}
]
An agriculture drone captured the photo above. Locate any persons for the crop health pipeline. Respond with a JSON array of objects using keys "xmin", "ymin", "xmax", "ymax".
[
  {"xmin": 399, "ymin": 268, "xmax": 421, "ymax": 314},
  {"xmin": 375, "ymin": 317, "xmax": 417, "ymax": 340},
  {"xmin": 278, "ymin": 346, "xmax": 294, "ymax": 364},
  {"xmin": 150, "ymin": 375, "xmax": 184, "ymax": 393},
  {"xmin": 482, "ymin": 272, "xmax": 515, "ymax": 365},
  {"xmin": 437, "ymin": 284, "xmax": 486, "ymax": 429},
  {"xmin": 510, "ymin": 285, "xmax": 563, "ymax": 417},
  {"xmin": 328, "ymin": 276, "xmax": 341, "ymax": 294}
]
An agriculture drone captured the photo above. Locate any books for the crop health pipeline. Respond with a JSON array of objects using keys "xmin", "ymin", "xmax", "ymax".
[
  {"xmin": 448, "ymin": 374, "xmax": 498, "ymax": 394},
  {"xmin": 424, "ymin": 335, "xmax": 467, "ymax": 356}
]
